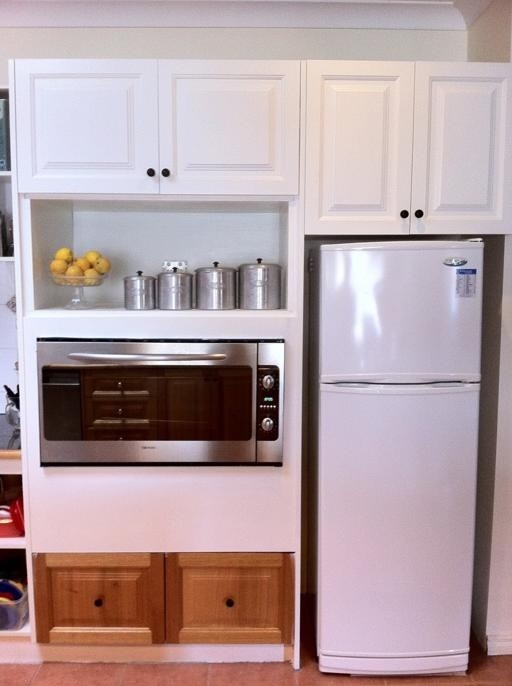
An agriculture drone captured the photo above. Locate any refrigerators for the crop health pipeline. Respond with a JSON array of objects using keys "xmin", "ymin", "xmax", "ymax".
[{"xmin": 305, "ymin": 236, "xmax": 484, "ymax": 674}]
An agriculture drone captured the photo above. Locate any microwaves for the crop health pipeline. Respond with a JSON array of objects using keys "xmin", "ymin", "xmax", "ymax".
[{"xmin": 35, "ymin": 334, "xmax": 286, "ymax": 466}]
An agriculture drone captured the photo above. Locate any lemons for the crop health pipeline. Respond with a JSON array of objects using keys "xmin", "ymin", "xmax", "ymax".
[{"xmin": 51, "ymin": 246, "xmax": 110, "ymax": 285}]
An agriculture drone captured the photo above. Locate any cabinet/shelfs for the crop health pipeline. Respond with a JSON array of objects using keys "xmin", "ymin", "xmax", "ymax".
[
  {"xmin": 12, "ymin": 54, "xmax": 304, "ymax": 200},
  {"xmin": 32, "ymin": 551, "xmax": 298, "ymax": 646},
  {"xmin": 297, "ymin": 59, "xmax": 511, "ymax": 236}
]
